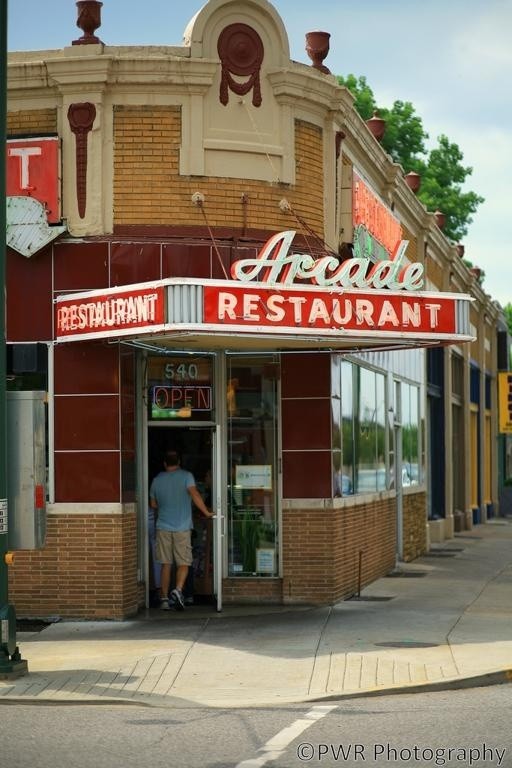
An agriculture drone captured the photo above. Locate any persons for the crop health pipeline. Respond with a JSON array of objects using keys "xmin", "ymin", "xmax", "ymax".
[
  {"xmin": 333, "ymin": 452, "xmax": 343, "ymax": 498},
  {"xmin": 373, "ymin": 448, "xmax": 412, "ymax": 491},
  {"xmin": 148, "ymin": 448, "xmax": 237, "ymax": 611},
  {"xmin": 342, "ymin": 453, "xmax": 353, "ymax": 495}
]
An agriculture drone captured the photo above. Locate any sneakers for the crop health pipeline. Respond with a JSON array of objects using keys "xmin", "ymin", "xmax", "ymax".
[{"xmin": 160, "ymin": 589, "xmax": 193, "ymax": 611}]
